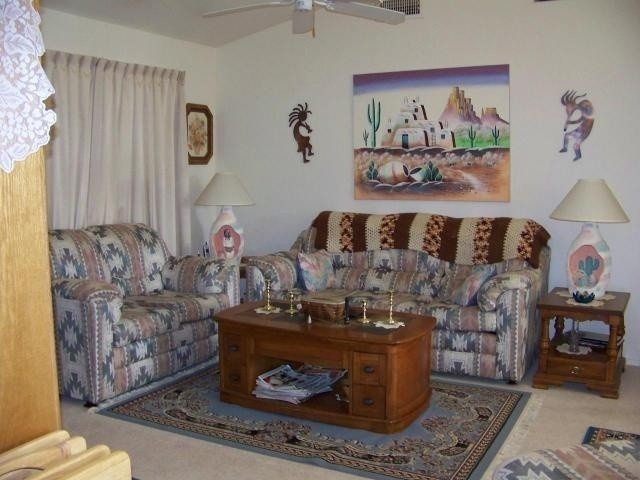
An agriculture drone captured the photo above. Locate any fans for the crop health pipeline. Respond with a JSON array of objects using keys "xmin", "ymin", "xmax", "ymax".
[{"xmin": 202, "ymin": 0, "xmax": 405, "ymax": 34}]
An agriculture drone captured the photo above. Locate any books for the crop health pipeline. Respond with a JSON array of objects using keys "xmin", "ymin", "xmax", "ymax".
[{"xmin": 251, "ymin": 364, "xmax": 348, "ymax": 405}]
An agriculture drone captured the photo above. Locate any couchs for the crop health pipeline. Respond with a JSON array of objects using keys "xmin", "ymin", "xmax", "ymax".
[{"xmin": 246, "ymin": 212, "xmax": 549, "ymax": 383}]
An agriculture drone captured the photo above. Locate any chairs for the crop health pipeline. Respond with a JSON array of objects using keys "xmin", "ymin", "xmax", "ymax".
[
  {"xmin": 491, "ymin": 443, "xmax": 640, "ymax": 480},
  {"xmin": 48, "ymin": 223, "xmax": 229, "ymax": 404}
]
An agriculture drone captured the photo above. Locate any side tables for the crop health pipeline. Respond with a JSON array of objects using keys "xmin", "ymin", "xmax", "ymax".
[{"xmin": 535, "ymin": 286, "xmax": 630, "ymax": 400}]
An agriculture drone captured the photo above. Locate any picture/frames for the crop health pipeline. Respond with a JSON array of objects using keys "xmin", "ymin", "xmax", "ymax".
[{"xmin": 186, "ymin": 103, "xmax": 213, "ymax": 164}]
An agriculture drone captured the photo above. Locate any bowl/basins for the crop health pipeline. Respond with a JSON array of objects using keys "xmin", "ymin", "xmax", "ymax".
[{"xmin": 345, "ymin": 295, "xmax": 372, "ymax": 317}]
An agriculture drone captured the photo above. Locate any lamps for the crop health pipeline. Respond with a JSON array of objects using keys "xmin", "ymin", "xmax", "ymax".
[
  {"xmin": 196, "ymin": 172, "xmax": 255, "ymax": 307},
  {"xmin": 550, "ymin": 179, "xmax": 631, "ymax": 299},
  {"xmin": 295, "ymin": 0, "xmax": 312, "ymax": 12}
]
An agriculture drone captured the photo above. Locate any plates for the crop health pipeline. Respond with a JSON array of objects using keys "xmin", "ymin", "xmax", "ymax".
[{"xmin": 573, "ymin": 291, "xmax": 595, "ymax": 303}]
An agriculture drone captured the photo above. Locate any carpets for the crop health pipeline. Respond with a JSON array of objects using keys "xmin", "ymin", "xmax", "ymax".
[
  {"xmin": 582, "ymin": 426, "xmax": 639, "ymax": 444},
  {"xmin": 96, "ymin": 360, "xmax": 532, "ymax": 480}
]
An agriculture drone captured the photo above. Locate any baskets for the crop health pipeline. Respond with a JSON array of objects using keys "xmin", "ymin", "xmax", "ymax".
[{"xmin": 299, "ymin": 299, "xmax": 347, "ymax": 323}]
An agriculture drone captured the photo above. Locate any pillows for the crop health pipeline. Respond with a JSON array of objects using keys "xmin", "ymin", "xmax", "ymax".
[
  {"xmin": 438, "ymin": 264, "xmax": 493, "ymax": 305},
  {"xmin": 297, "ymin": 248, "xmax": 334, "ymax": 292}
]
